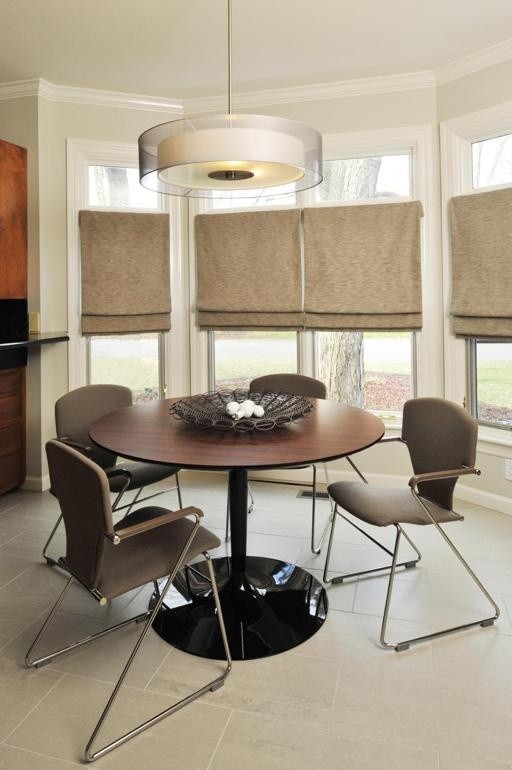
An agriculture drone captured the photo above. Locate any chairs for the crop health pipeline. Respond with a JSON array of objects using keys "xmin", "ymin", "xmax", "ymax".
[
  {"xmin": 225, "ymin": 374, "xmax": 334, "ymax": 554},
  {"xmin": 323, "ymin": 397, "xmax": 499, "ymax": 651},
  {"xmin": 43, "ymin": 384, "xmax": 183, "ymax": 567},
  {"xmin": 23, "ymin": 439, "xmax": 232, "ymax": 764}
]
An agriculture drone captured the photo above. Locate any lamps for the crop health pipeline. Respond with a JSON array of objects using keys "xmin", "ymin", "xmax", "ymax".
[{"xmin": 139, "ymin": 0, "xmax": 322, "ymax": 198}]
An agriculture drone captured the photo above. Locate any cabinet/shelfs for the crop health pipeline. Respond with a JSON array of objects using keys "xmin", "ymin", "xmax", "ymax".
[
  {"xmin": 0, "ymin": 140, "xmax": 27, "ymax": 298},
  {"xmin": 0, "ymin": 366, "xmax": 27, "ymax": 496}
]
